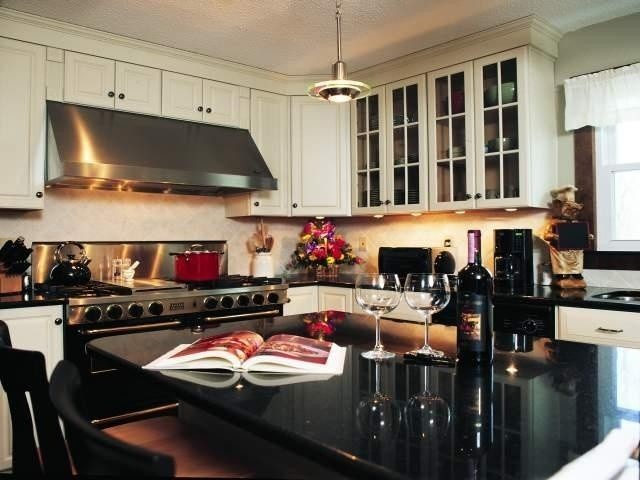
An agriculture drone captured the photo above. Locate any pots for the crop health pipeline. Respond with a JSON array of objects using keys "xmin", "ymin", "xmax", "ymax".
[{"xmin": 168, "ymin": 243, "xmax": 224, "ymax": 279}]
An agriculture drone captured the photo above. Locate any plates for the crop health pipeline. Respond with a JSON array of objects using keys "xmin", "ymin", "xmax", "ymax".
[
  {"xmin": 362, "ymin": 110, "xmax": 419, "ymax": 206},
  {"xmin": 434, "ymin": 251, "xmax": 455, "ymax": 273}
]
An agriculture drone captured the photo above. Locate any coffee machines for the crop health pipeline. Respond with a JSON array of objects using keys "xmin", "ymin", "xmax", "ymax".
[{"xmin": 494, "ymin": 228, "xmax": 533, "ymax": 293}]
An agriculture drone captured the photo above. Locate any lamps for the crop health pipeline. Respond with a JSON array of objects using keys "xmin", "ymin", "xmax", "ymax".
[{"xmin": 306, "ymin": 0, "xmax": 371, "ymax": 104}]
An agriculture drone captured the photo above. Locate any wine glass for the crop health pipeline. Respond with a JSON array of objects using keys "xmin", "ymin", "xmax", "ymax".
[
  {"xmin": 406, "ymin": 363, "xmax": 449, "ymax": 440},
  {"xmin": 356, "ymin": 356, "xmax": 402, "ymax": 443},
  {"xmin": 355, "ymin": 272, "xmax": 402, "ymax": 360},
  {"xmin": 404, "ymin": 272, "xmax": 451, "ymax": 358}
]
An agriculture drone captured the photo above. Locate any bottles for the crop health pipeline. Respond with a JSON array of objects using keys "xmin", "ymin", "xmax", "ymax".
[
  {"xmin": 112, "ymin": 259, "xmax": 122, "ymax": 279},
  {"xmin": 251, "ymin": 253, "xmax": 275, "ymax": 278},
  {"xmin": 459, "ymin": 365, "xmax": 492, "ymax": 479},
  {"xmin": 457, "ymin": 229, "xmax": 494, "ymax": 364},
  {"xmin": 121, "ymin": 258, "xmax": 131, "ymax": 279}
]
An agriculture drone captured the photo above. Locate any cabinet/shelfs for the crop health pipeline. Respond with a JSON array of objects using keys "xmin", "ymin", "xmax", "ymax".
[
  {"xmin": 282, "ymin": 284, "xmax": 318, "ymax": 318},
  {"xmin": 0, "ymin": 34, "xmax": 47, "ymax": 212},
  {"xmin": 226, "ymin": 88, "xmax": 351, "ymax": 218},
  {"xmin": 63, "ymin": 50, "xmax": 251, "ymax": 132},
  {"xmin": 351, "ymin": 42, "xmax": 558, "ymax": 219},
  {"xmin": 317, "ymin": 285, "xmax": 353, "ymax": 313},
  {"xmin": 0, "ymin": 305, "xmax": 65, "ymax": 475},
  {"xmin": 554, "ymin": 303, "xmax": 640, "ymax": 349},
  {"xmin": 353, "ymin": 289, "xmax": 432, "ymax": 323}
]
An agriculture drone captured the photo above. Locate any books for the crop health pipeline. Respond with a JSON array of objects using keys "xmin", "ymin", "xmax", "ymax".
[
  {"xmin": 156, "ymin": 369, "xmax": 335, "ymax": 390},
  {"xmin": 140, "ymin": 329, "xmax": 347, "ymax": 375}
]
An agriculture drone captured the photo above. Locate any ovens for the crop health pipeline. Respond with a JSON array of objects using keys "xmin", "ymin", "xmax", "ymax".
[
  {"xmin": 201, "ymin": 303, "xmax": 284, "ymax": 326},
  {"xmin": 69, "ymin": 314, "xmax": 193, "ymax": 428}
]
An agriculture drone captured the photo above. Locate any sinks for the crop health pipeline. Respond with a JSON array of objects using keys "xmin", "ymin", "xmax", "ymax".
[{"xmin": 598, "ymin": 291, "xmax": 640, "ymax": 302}]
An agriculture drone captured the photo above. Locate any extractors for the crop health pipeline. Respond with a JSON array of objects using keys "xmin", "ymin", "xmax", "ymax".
[{"xmin": 46, "ymin": 100, "xmax": 278, "ymax": 197}]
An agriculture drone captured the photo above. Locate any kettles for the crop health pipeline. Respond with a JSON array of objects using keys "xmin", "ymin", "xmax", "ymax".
[{"xmin": 50, "ymin": 242, "xmax": 93, "ymax": 283}]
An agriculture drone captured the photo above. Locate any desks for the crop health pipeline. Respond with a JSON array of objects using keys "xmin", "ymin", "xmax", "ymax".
[{"xmin": 82, "ymin": 310, "xmax": 638, "ymax": 480}]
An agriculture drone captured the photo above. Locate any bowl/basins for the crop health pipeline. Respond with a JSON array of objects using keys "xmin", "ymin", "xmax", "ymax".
[
  {"xmin": 440, "ymin": 80, "xmax": 524, "ymax": 201},
  {"xmin": 123, "ymin": 270, "xmax": 136, "ymax": 280}
]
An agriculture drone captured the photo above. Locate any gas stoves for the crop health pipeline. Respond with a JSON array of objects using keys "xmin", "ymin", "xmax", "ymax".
[
  {"xmin": 36, "ymin": 280, "xmax": 131, "ymax": 296},
  {"xmin": 181, "ymin": 274, "xmax": 282, "ymax": 288}
]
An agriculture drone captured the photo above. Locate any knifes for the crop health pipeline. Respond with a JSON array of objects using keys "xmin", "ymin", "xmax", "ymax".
[{"xmin": 0, "ymin": 236, "xmax": 32, "ymax": 293}]
